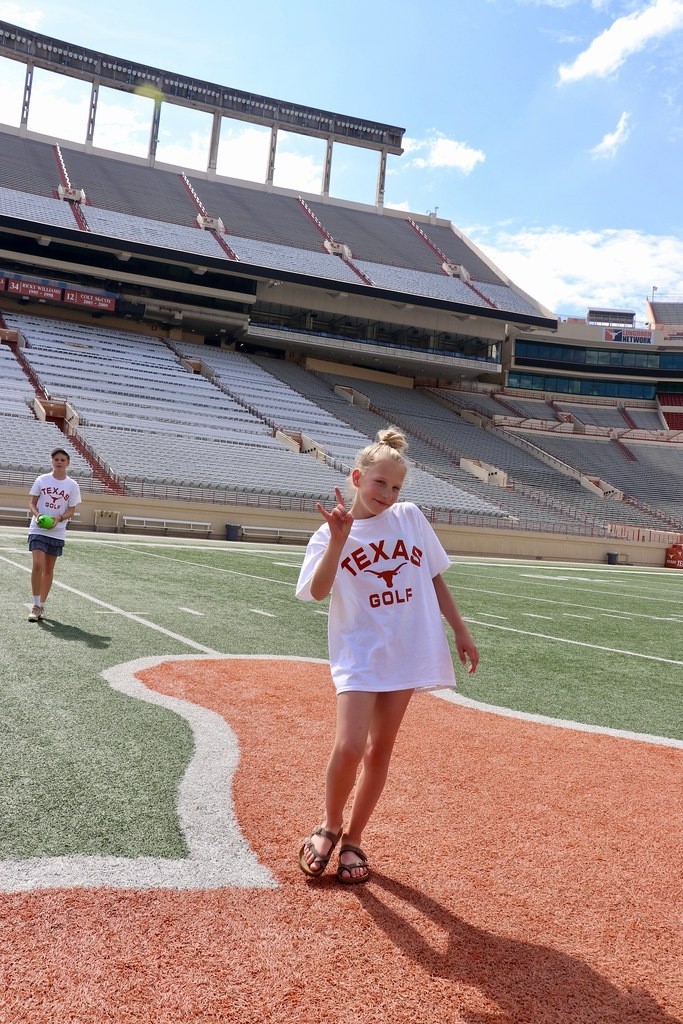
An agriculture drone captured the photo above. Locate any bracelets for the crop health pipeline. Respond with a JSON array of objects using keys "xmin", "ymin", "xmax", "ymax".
[{"xmin": 60, "ymin": 514, "xmax": 63, "ymax": 523}]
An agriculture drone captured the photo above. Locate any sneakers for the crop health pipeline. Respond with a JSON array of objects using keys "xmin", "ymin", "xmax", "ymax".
[
  {"xmin": 38, "ymin": 607, "xmax": 46, "ymax": 618},
  {"xmin": 27, "ymin": 605, "xmax": 41, "ymax": 621}
]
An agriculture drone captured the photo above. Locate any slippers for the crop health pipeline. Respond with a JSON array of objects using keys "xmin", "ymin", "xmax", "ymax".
[
  {"xmin": 337, "ymin": 844, "xmax": 369, "ymax": 883},
  {"xmin": 298, "ymin": 825, "xmax": 343, "ymax": 876}
]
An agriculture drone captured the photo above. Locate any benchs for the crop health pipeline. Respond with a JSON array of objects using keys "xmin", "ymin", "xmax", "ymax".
[
  {"xmin": 241, "ymin": 526, "xmax": 316, "ymax": 545},
  {"xmin": 123, "ymin": 516, "xmax": 213, "ymax": 540},
  {"xmin": 1, "ymin": 507, "xmax": 82, "ymax": 529}
]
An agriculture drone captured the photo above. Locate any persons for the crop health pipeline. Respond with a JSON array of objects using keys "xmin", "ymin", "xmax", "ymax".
[
  {"xmin": 295, "ymin": 427, "xmax": 479, "ymax": 882},
  {"xmin": 27, "ymin": 448, "xmax": 81, "ymax": 622}
]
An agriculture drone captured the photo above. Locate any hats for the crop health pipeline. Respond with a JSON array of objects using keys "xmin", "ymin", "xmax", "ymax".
[{"xmin": 50, "ymin": 447, "xmax": 70, "ymax": 460}]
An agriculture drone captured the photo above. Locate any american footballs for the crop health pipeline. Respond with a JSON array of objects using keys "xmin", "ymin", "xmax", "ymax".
[{"xmin": 38, "ymin": 515, "xmax": 54, "ymax": 529}]
